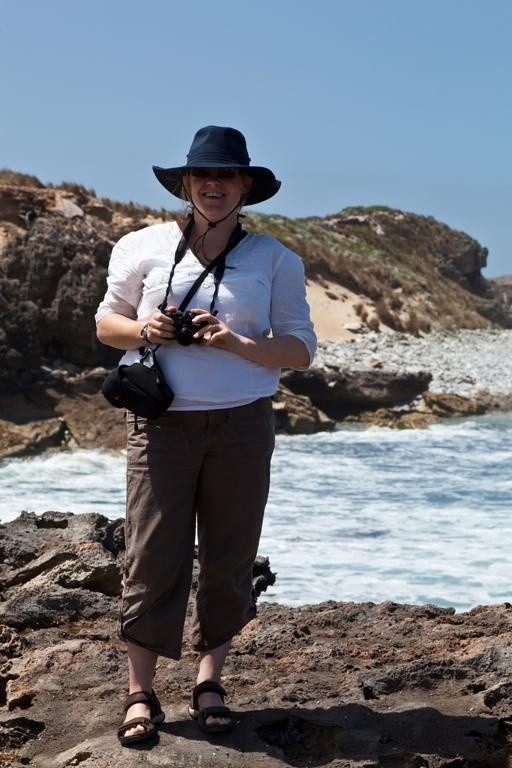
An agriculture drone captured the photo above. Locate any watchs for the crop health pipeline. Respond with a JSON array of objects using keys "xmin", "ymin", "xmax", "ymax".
[{"xmin": 140, "ymin": 322, "xmax": 151, "ymax": 345}]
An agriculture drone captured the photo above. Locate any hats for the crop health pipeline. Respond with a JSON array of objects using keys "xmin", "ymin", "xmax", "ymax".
[{"xmin": 151, "ymin": 123, "xmax": 284, "ymax": 207}]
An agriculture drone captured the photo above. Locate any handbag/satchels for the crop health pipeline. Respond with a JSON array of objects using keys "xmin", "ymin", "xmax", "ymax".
[{"xmin": 99, "ymin": 348, "xmax": 176, "ymax": 423}]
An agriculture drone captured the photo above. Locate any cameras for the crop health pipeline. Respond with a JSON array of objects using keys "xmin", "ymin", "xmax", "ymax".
[{"xmin": 162, "ymin": 309, "xmax": 209, "ymax": 348}]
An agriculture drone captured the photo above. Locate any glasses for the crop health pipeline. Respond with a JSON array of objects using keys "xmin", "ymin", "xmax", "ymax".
[{"xmin": 190, "ymin": 167, "xmax": 246, "ymax": 179}]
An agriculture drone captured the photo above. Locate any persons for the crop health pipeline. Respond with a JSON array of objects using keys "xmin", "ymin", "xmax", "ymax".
[{"xmin": 94, "ymin": 124, "xmax": 319, "ymax": 744}]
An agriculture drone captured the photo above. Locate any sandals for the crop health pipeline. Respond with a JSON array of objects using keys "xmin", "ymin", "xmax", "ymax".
[
  {"xmin": 116, "ymin": 684, "xmax": 167, "ymax": 748},
  {"xmin": 185, "ymin": 678, "xmax": 237, "ymax": 735}
]
